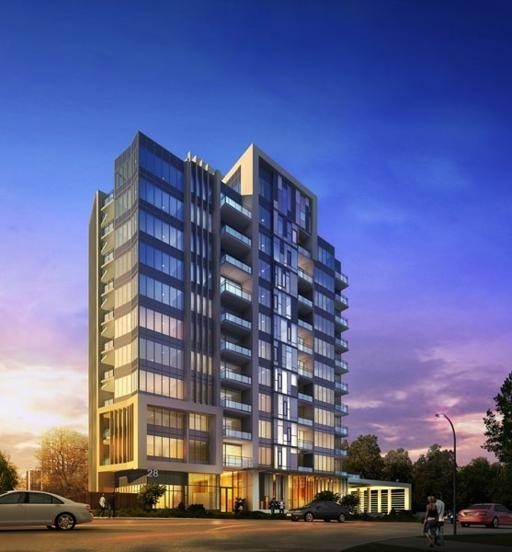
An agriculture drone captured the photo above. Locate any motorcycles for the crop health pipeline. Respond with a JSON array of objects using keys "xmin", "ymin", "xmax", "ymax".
[{"xmin": 233, "ymin": 498, "xmax": 246, "ymax": 515}]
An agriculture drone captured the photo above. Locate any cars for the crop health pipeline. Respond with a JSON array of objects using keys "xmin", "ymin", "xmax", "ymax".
[
  {"xmin": 0, "ymin": 489, "xmax": 93, "ymax": 531},
  {"xmin": 457, "ymin": 502, "xmax": 512, "ymax": 528},
  {"xmin": 286, "ymin": 500, "xmax": 343, "ymax": 524}
]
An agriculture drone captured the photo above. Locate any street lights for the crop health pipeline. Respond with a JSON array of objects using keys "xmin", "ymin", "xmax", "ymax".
[{"xmin": 435, "ymin": 412, "xmax": 457, "ymax": 537}]
